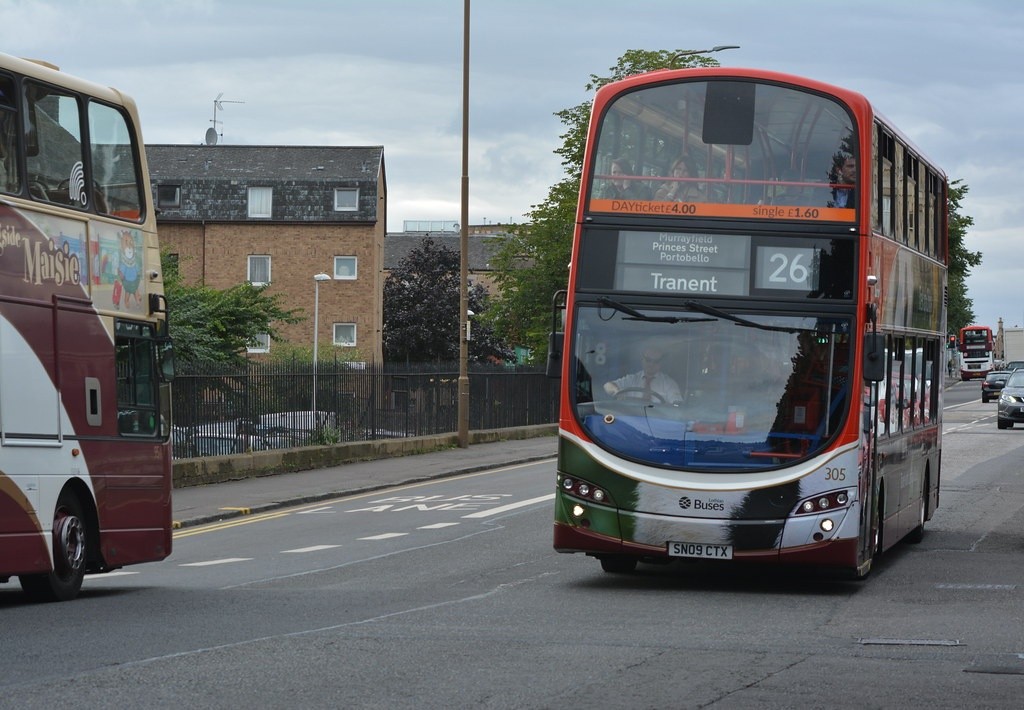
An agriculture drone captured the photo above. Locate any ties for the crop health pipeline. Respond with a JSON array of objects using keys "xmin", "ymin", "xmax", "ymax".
[{"xmin": 642, "ymin": 377, "xmax": 655, "ymax": 401}]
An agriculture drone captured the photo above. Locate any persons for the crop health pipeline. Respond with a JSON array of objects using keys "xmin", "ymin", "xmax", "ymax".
[
  {"xmin": 811, "ymin": 149, "xmax": 861, "ymax": 206},
  {"xmin": 604, "ymin": 346, "xmax": 683, "ymax": 406},
  {"xmin": 596, "ymin": 157, "xmax": 652, "ymax": 203},
  {"xmin": 947, "ymin": 359, "xmax": 952, "ymax": 377},
  {"xmin": 652, "ymin": 154, "xmax": 708, "ymax": 201}
]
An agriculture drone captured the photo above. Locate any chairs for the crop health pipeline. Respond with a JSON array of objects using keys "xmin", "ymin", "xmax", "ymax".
[
  {"xmin": 20, "ymin": 175, "xmax": 110, "ymax": 215},
  {"xmin": 639, "ymin": 337, "xmax": 686, "ymax": 400}
]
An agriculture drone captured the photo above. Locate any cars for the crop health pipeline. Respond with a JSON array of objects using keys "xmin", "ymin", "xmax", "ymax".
[
  {"xmin": 981, "ymin": 371, "xmax": 1011, "ymax": 402},
  {"xmin": 997, "ymin": 368, "xmax": 1024, "ymax": 429},
  {"xmin": 1003, "ymin": 361, "xmax": 1024, "ymax": 371},
  {"xmin": 171, "ymin": 409, "xmax": 341, "ymax": 460}
]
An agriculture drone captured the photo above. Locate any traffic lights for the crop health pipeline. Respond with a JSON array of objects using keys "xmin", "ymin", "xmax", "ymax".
[
  {"xmin": 946, "ymin": 334, "xmax": 949, "ymax": 349},
  {"xmin": 950, "ymin": 335, "xmax": 955, "ymax": 348}
]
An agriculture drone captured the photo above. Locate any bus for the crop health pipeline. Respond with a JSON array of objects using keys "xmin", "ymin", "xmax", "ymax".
[
  {"xmin": 959, "ymin": 326, "xmax": 994, "ymax": 381},
  {"xmin": 1, "ymin": 52, "xmax": 174, "ymax": 598},
  {"xmin": 546, "ymin": 67, "xmax": 949, "ymax": 581}
]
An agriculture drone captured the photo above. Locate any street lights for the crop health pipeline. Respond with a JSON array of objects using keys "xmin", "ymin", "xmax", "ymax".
[
  {"xmin": 668, "ymin": 45, "xmax": 740, "ymax": 69},
  {"xmin": 312, "ymin": 274, "xmax": 331, "ymax": 411}
]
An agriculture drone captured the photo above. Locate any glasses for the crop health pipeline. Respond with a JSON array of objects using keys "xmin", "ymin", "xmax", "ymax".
[{"xmin": 643, "ymin": 355, "xmax": 663, "ymax": 363}]
